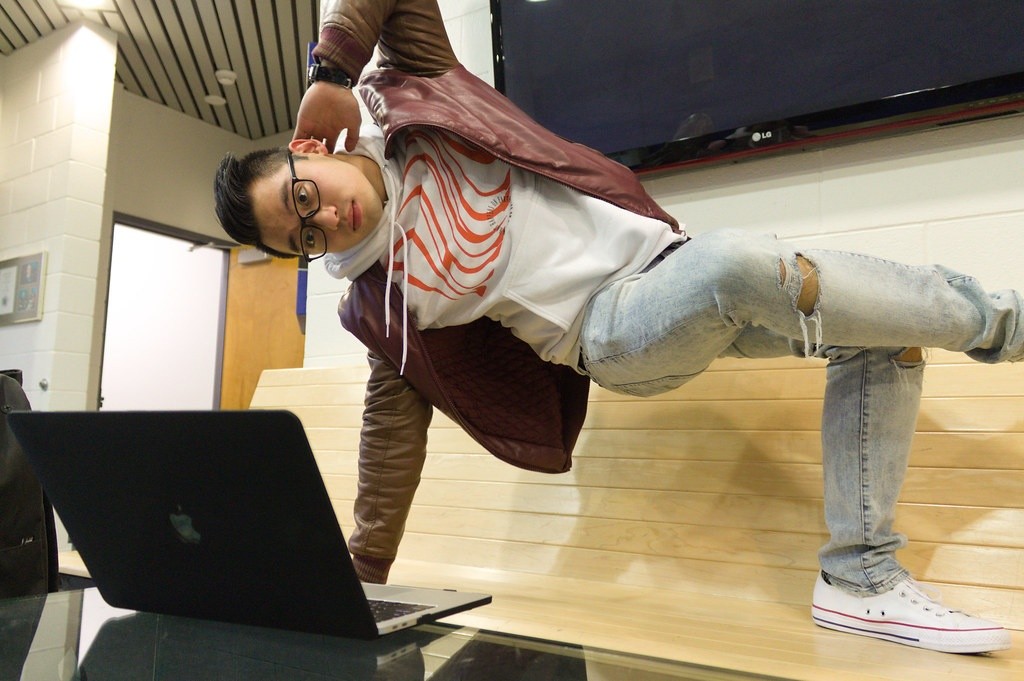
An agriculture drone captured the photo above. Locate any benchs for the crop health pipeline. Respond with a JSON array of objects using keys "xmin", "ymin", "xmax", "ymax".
[{"xmin": 57, "ymin": 353, "xmax": 1024, "ymax": 681}]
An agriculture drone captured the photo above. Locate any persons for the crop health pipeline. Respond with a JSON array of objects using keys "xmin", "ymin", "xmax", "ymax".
[{"xmin": 212, "ymin": 0, "xmax": 1024, "ymax": 655}]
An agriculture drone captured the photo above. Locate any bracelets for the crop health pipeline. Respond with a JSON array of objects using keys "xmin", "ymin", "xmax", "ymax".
[{"xmin": 309, "ymin": 63, "xmax": 356, "ymax": 89}]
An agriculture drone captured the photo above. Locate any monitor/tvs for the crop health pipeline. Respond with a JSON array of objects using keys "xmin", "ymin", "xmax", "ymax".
[{"xmin": 487, "ymin": 0, "xmax": 1024, "ymax": 176}]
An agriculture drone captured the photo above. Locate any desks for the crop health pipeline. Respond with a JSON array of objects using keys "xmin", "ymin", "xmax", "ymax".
[{"xmin": 0, "ymin": 566, "xmax": 794, "ymax": 681}]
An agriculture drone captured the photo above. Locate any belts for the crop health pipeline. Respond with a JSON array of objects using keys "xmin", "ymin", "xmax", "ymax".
[{"xmin": 640, "ymin": 241, "xmax": 685, "ymax": 274}]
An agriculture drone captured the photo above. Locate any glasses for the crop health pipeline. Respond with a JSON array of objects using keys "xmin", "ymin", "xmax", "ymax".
[{"xmin": 287, "ymin": 148, "xmax": 328, "ymax": 262}]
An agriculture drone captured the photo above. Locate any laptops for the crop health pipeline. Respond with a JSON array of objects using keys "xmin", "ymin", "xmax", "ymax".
[{"xmin": 4, "ymin": 407, "xmax": 492, "ymax": 644}]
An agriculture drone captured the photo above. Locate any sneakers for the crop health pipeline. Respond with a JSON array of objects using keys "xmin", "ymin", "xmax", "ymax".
[{"xmin": 812, "ymin": 569, "xmax": 1011, "ymax": 654}]
